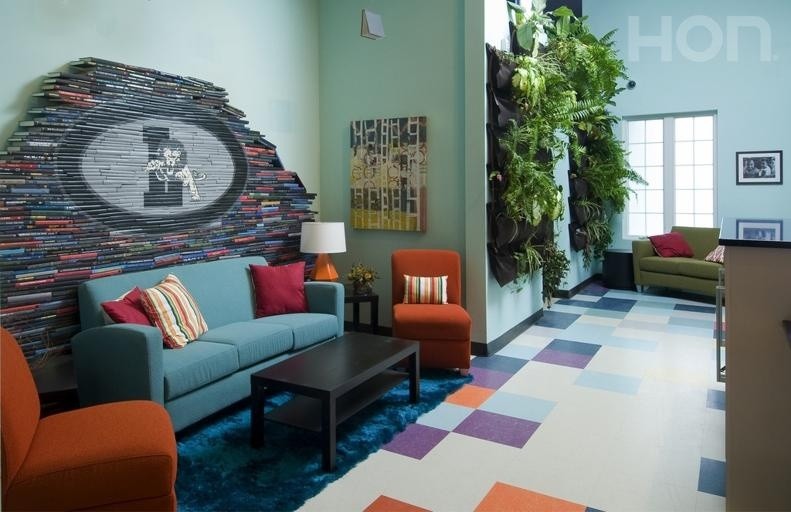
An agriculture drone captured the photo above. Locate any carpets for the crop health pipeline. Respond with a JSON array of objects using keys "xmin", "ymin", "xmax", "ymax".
[{"xmin": 173, "ymin": 367, "xmax": 475, "ymax": 512}]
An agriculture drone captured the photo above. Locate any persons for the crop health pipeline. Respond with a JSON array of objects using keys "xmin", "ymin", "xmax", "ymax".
[
  {"xmin": 758, "ymin": 160, "xmax": 773, "ymax": 177},
  {"xmin": 742, "ymin": 158, "xmax": 758, "ymax": 178}
]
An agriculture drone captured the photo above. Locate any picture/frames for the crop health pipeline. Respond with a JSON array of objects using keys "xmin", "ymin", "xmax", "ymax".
[
  {"xmin": 735, "ymin": 219, "xmax": 784, "ymax": 243},
  {"xmin": 735, "ymin": 150, "xmax": 783, "ymax": 186},
  {"xmin": 349, "ymin": 115, "xmax": 428, "ymax": 234}
]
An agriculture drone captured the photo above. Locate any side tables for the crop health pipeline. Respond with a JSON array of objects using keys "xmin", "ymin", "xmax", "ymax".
[{"xmin": 344, "ymin": 285, "xmax": 379, "ymax": 336}]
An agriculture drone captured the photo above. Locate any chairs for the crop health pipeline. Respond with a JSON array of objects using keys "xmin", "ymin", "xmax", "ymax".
[{"xmin": 388, "ymin": 248, "xmax": 473, "ymax": 379}]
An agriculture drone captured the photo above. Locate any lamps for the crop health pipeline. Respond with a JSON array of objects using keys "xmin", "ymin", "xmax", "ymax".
[{"xmin": 300, "ymin": 220, "xmax": 349, "ymax": 281}]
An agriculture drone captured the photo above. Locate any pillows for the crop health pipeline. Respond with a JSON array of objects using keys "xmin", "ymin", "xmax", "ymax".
[
  {"xmin": 402, "ymin": 273, "xmax": 449, "ymax": 305},
  {"xmin": 101, "ymin": 285, "xmax": 154, "ymax": 328},
  {"xmin": 248, "ymin": 261, "xmax": 309, "ymax": 319},
  {"xmin": 647, "ymin": 230, "xmax": 693, "ymax": 258},
  {"xmin": 141, "ymin": 272, "xmax": 208, "ymax": 349}
]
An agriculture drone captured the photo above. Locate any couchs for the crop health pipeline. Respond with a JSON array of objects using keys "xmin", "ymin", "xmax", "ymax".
[
  {"xmin": 1, "ymin": 326, "xmax": 178, "ymax": 511},
  {"xmin": 69, "ymin": 256, "xmax": 348, "ymax": 434},
  {"xmin": 632, "ymin": 226, "xmax": 725, "ymax": 299}
]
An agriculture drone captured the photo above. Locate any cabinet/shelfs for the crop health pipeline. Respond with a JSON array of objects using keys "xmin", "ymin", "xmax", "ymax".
[{"xmin": 716, "ymin": 239, "xmax": 791, "ymax": 511}]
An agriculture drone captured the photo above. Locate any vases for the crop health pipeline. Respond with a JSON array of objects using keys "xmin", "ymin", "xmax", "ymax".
[{"xmin": 354, "ymin": 281, "xmax": 368, "ymax": 294}]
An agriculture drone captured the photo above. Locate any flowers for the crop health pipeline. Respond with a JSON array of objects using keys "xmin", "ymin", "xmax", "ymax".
[{"xmin": 347, "ymin": 262, "xmax": 384, "ymax": 287}]
